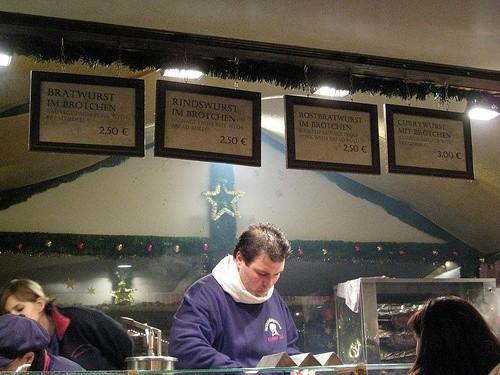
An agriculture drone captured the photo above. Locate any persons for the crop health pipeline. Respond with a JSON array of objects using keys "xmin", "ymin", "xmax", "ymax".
[
  {"xmin": 0, "ymin": 277, "xmax": 134, "ymax": 370},
  {"xmin": 407, "ymin": 295, "xmax": 500, "ymax": 375},
  {"xmin": 168, "ymin": 222, "xmax": 303, "ymax": 369},
  {"xmin": 0, "ymin": 312, "xmax": 86, "ymax": 372}
]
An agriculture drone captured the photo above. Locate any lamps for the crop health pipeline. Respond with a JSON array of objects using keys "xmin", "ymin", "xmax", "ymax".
[{"xmin": 464, "ymin": 93, "xmax": 500, "ymax": 120}]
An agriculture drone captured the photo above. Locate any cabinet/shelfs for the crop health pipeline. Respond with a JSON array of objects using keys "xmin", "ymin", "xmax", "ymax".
[{"xmin": 333, "ymin": 277, "xmax": 496, "ymax": 364}]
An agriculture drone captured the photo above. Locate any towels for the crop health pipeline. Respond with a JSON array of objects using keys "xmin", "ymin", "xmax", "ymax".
[
  {"xmin": 336, "ymin": 276, "xmax": 388, "ymax": 313},
  {"xmin": 212, "ymin": 255, "xmax": 274, "ymax": 304}
]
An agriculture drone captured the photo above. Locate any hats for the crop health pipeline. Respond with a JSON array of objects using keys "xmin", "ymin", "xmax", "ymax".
[{"xmin": 0, "ymin": 313, "xmax": 51, "ymax": 367}]
